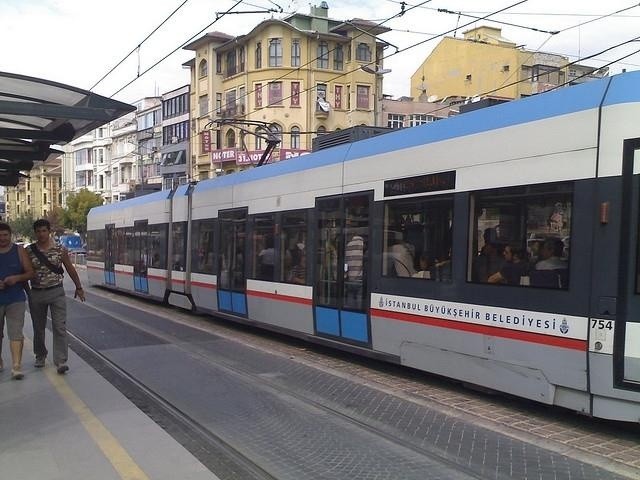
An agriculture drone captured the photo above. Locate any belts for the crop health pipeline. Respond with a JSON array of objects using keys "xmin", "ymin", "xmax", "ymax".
[{"xmin": 31, "ymin": 283, "xmax": 63, "ymax": 291}]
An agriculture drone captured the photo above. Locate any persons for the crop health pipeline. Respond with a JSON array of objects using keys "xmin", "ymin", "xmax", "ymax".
[
  {"xmin": 0, "ymin": 222, "xmax": 36, "ymax": 378},
  {"xmin": 22, "ymin": 219, "xmax": 86, "ymax": 374},
  {"xmin": 247, "ymin": 220, "xmax": 454, "ymax": 311},
  {"xmin": 474, "ymin": 228, "xmax": 570, "ymax": 291}
]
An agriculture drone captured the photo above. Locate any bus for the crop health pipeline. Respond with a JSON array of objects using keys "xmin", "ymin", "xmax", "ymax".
[{"xmin": 83, "ymin": 62, "xmax": 640, "ymax": 433}]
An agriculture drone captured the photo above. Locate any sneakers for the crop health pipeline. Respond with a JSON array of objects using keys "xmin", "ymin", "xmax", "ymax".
[
  {"xmin": 32, "ymin": 350, "xmax": 48, "ymax": 368},
  {"xmin": 56, "ymin": 364, "xmax": 69, "ymax": 374},
  {"xmin": 8, "ymin": 369, "xmax": 26, "ymax": 379}
]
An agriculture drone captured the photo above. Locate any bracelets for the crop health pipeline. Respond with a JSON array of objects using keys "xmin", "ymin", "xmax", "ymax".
[{"xmin": 76, "ymin": 287, "xmax": 83, "ymax": 290}]
